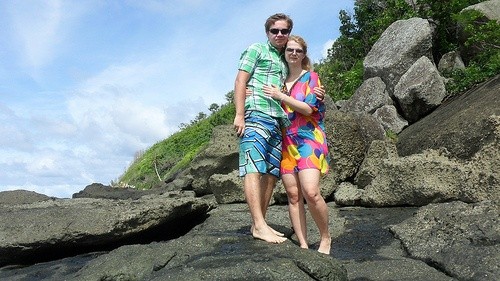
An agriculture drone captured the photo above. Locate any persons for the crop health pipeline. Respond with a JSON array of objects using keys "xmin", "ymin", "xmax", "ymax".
[
  {"xmin": 233, "ymin": 11, "xmax": 326, "ymax": 243},
  {"xmin": 243, "ymin": 34, "xmax": 332, "ymax": 255}
]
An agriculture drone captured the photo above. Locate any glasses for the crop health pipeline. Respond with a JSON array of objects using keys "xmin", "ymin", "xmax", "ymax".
[
  {"xmin": 268, "ymin": 29, "xmax": 290, "ymax": 34},
  {"xmin": 286, "ymin": 48, "xmax": 304, "ymax": 53}
]
